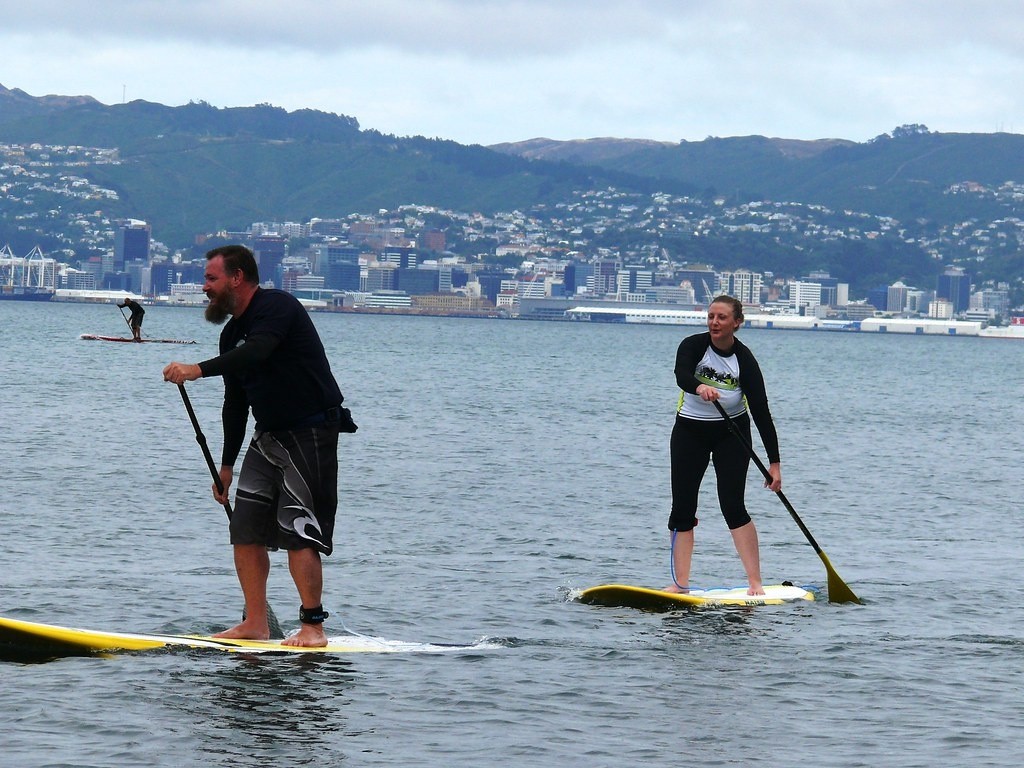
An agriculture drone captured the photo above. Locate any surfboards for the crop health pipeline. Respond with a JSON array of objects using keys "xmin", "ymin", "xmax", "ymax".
[
  {"xmin": 78, "ymin": 334, "xmax": 198, "ymax": 345},
  {"xmin": 1, "ymin": 616, "xmax": 509, "ymax": 662},
  {"xmin": 579, "ymin": 583, "xmax": 815, "ymax": 610}
]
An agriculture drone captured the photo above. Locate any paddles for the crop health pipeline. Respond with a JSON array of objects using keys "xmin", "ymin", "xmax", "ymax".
[
  {"xmin": 119, "ymin": 306, "xmax": 133, "ymax": 333},
  {"xmin": 163, "ymin": 359, "xmax": 291, "ymax": 638},
  {"xmin": 712, "ymin": 396, "xmax": 861, "ymax": 606}
]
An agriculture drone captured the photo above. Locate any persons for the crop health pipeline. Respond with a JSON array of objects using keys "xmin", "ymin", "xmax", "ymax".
[
  {"xmin": 657, "ymin": 295, "xmax": 784, "ymax": 596},
  {"xmin": 116, "ymin": 298, "xmax": 146, "ymax": 343},
  {"xmin": 161, "ymin": 245, "xmax": 359, "ymax": 649}
]
